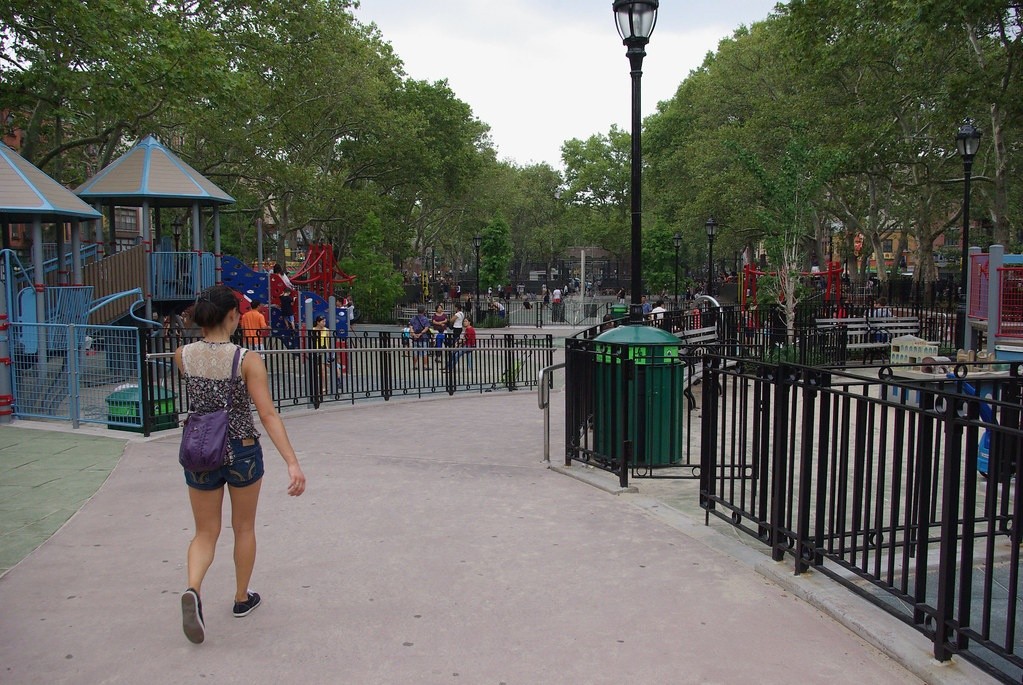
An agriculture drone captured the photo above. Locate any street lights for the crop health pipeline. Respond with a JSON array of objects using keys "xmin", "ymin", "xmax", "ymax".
[
  {"xmin": 671, "ymin": 232, "xmax": 682, "ymax": 323},
  {"xmin": 473, "ymin": 233, "xmax": 483, "ymax": 322},
  {"xmin": 827, "ymin": 226, "xmax": 834, "ymax": 263},
  {"xmin": 431, "ymin": 245, "xmax": 436, "ymax": 281},
  {"xmin": 954, "ymin": 118, "xmax": 982, "ymax": 351},
  {"xmin": 612, "ymin": 0, "xmax": 660, "ymax": 326},
  {"xmin": 703, "ymin": 218, "xmax": 719, "ymax": 309}
]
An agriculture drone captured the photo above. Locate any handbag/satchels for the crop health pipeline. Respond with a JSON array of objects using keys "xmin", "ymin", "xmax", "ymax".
[
  {"xmin": 179, "ymin": 348, "xmax": 241, "ymax": 473},
  {"xmin": 426, "ymin": 329, "xmax": 431, "ymax": 339}
]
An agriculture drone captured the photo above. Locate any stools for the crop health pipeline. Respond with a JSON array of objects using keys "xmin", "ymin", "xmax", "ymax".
[{"xmin": 465, "ymin": 346, "xmax": 476, "ymax": 370}]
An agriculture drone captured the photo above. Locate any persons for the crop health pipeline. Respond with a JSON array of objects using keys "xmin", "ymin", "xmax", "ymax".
[
  {"xmin": 391, "ymin": 259, "xmax": 908, "ymax": 372},
  {"xmin": 241, "ymin": 262, "xmax": 355, "ymax": 390},
  {"xmin": 175, "ymin": 285, "xmax": 306, "ymax": 643}
]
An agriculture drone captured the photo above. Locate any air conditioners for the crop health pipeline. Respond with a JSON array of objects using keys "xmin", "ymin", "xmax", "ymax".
[{"xmin": 13, "ymin": 233, "xmax": 18, "ymax": 238}]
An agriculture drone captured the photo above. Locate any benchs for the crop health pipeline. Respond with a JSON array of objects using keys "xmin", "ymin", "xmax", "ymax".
[
  {"xmin": 815, "ymin": 316, "xmax": 936, "ymax": 365},
  {"xmin": 396, "ymin": 308, "xmax": 452, "ymax": 327},
  {"xmin": 674, "ymin": 326, "xmax": 739, "ymax": 410}
]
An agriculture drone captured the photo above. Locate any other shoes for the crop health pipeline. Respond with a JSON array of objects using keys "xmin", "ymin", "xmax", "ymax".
[
  {"xmin": 413, "ymin": 367, "xmax": 418, "ymax": 370},
  {"xmin": 424, "ymin": 367, "xmax": 432, "ymax": 370},
  {"xmin": 232, "ymin": 592, "xmax": 261, "ymax": 618},
  {"xmin": 181, "ymin": 588, "xmax": 205, "ymax": 645},
  {"xmin": 438, "ymin": 367, "xmax": 451, "ymax": 373}
]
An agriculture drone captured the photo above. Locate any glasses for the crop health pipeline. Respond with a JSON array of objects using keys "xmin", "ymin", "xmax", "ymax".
[{"xmin": 316, "ymin": 316, "xmax": 325, "ymax": 321}]
